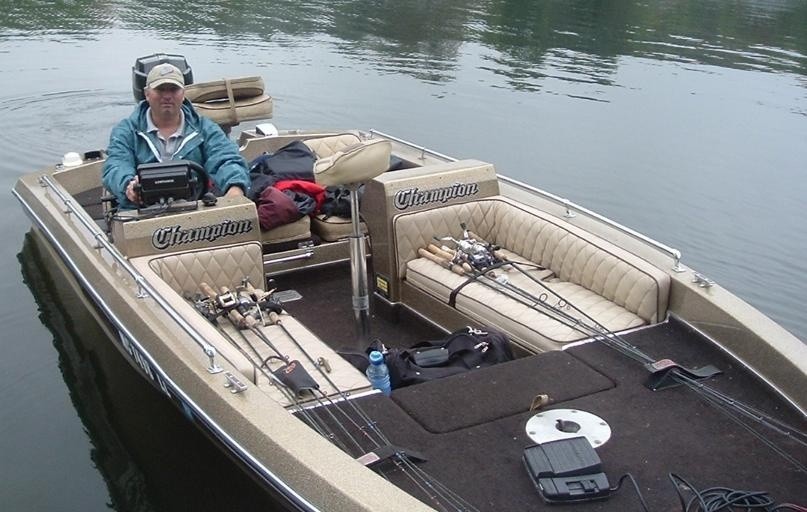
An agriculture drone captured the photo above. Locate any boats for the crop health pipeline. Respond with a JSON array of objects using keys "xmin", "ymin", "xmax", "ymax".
[{"xmin": 13, "ymin": 53, "xmax": 802, "ymax": 510}]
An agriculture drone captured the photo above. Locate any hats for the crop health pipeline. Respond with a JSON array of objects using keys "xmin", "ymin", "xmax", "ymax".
[{"xmin": 147, "ymin": 63, "xmax": 186, "ymax": 93}]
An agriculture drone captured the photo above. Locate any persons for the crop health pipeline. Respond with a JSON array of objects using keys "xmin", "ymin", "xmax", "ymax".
[{"xmin": 100, "ymin": 62, "xmax": 251, "ymax": 213}]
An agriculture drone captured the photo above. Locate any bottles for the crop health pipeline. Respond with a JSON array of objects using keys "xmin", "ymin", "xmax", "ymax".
[{"xmin": 365, "ymin": 350, "xmax": 392, "ymax": 398}]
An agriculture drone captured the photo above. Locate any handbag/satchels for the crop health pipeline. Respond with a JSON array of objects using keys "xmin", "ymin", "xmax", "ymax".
[{"xmin": 368, "ymin": 326, "xmax": 515, "ymax": 387}]
